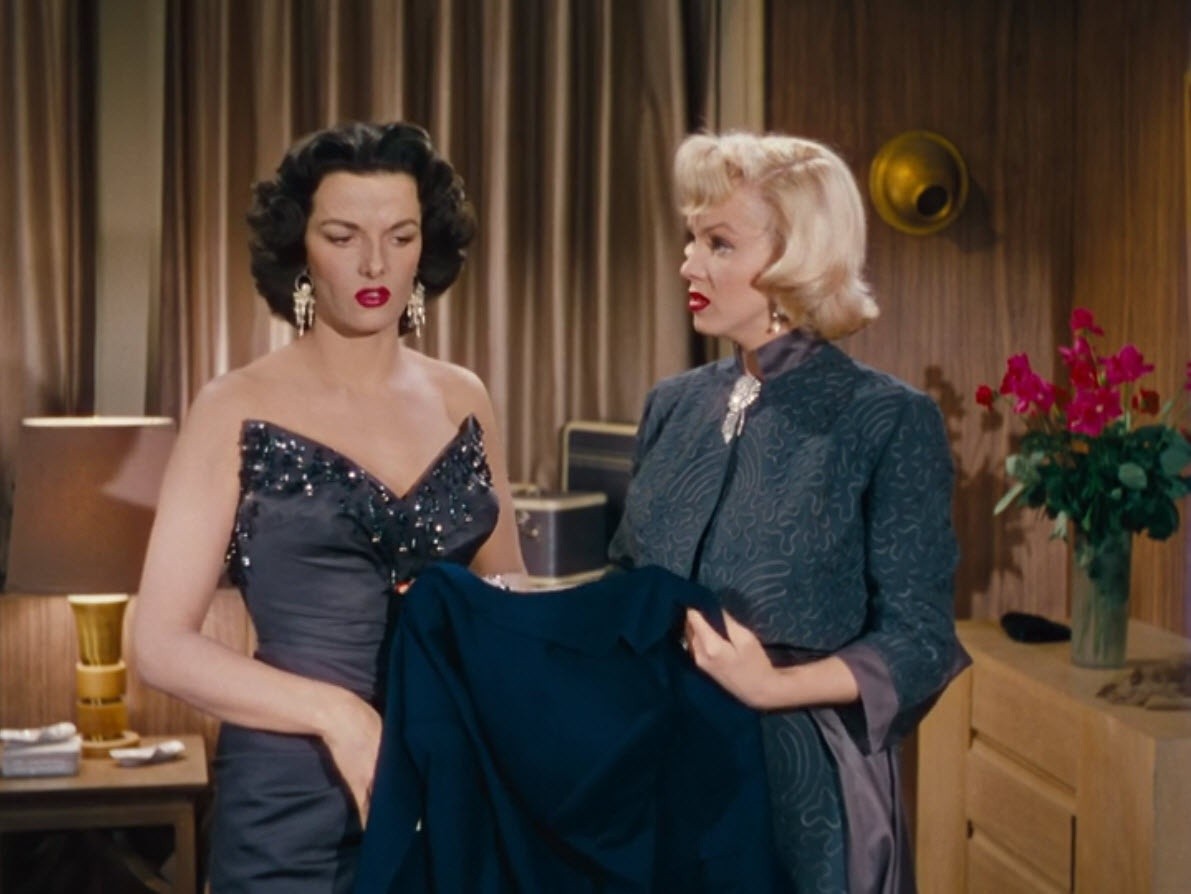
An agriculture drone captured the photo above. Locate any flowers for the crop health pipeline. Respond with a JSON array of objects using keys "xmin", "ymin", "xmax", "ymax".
[{"xmin": 976, "ymin": 306, "xmax": 1191, "ymax": 666}]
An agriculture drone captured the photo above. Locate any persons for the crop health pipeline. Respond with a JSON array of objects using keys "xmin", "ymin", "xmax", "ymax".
[
  {"xmin": 133, "ymin": 121, "xmax": 529, "ymax": 894},
  {"xmin": 605, "ymin": 130, "xmax": 972, "ymax": 894}
]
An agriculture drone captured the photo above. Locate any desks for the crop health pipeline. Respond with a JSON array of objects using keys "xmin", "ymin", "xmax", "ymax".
[{"xmin": 0, "ymin": 735, "xmax": 210, "ymax": 894}]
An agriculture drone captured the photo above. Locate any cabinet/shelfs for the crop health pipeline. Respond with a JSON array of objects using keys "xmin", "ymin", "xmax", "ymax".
[{"xmin": 913, "ymin": 614, "xmax": 1190, "ymax": 893}]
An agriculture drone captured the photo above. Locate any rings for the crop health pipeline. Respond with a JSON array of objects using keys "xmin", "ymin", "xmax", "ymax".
[{"xmin": 680, "ymin": 637, "xmax": 689, "ymax": 650}]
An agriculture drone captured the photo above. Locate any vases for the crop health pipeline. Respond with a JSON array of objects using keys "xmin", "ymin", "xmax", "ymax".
[{"xmin": 1071, "ymin": 526, "xmax": 1131, "ymax": 670}]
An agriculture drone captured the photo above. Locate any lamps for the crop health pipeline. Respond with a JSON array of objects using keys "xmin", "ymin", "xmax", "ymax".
[{"xmin": 6, "ymin": 416, "xmax": 177, "ymax": 758}]
